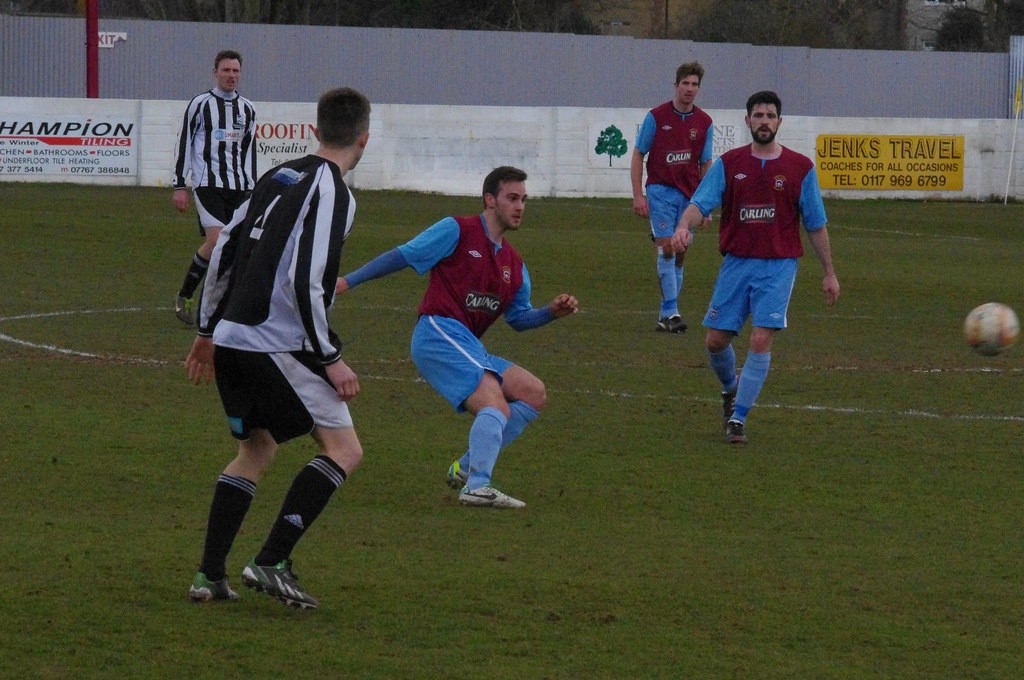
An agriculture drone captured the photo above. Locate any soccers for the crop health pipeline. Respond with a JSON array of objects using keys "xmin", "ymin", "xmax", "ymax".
[{"xmin": 962, "ymin": 303, "xmax": 1020, "ymax": 356}]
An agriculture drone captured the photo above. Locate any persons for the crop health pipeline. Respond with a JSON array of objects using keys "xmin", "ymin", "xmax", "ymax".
[
  {"xmin": 171, "ymin": 50, "xmax": 259, "ymax": 330},
  {"xmin": 183, "ymin": 88, "xmax": 372, "ymax": 609},
  {"xmin": 631, "ymin": 59, "xmax": 716, "ymax": 333},
  {"xmin": 336, "ymin": 165, "xmax": 580, "ymax": 508},
  {"xmin": 671, "ymin": 90, "xmax": 841, "ymax": 443}
]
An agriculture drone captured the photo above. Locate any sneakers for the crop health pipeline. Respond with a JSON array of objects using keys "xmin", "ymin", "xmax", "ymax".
[
  {"xmin": 725, "ymin": 420, "xmax": 744, "ymax": 443},
  {"xmin": 446, "ymin": 460, "xmax": 469, "ymax": 489},
  {"xmin": 668, "ymin": 313, "xmax": 688, "ymax": 333},
  {"xmin": 720, "ymin": 390, "xmax": 736, "ymax": 431},
  {"xmin": 175, "ymin": 288, "xmax": 194, "ymax": 326},
  {"xmin": 241, "ymin": 555, "xmax": 320, "ymax": 609},
  {"xmin": 188, "ymin": 571, "xmax": 243, "ymax": 600},
  {"xmin": 656, "ymin": 316, "xmax": 669, "ymax": 331},
  {"xmin": 457, "ymin": 484, "xmax": 526, "ymax": 509}
]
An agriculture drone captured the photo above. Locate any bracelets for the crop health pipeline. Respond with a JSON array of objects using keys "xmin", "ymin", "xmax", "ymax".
[{"xmin": 174, "ymin": 187, "xmax": 187, "ymax": 190}]
